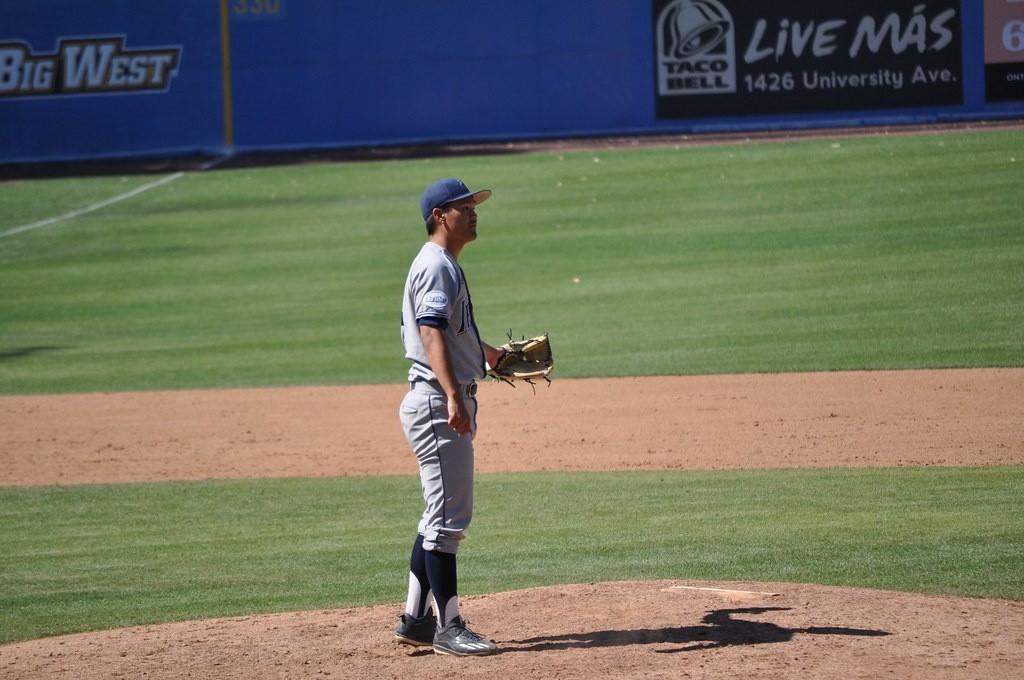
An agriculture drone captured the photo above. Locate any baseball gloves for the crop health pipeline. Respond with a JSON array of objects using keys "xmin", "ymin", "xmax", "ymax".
[{"xmin": 487, "ymin": 332, "xmax": 554, "ymax": 382}]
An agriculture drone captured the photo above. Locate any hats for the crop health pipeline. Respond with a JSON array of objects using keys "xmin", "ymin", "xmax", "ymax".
[{"xmin": 420, "ymin": 179, "xmax": 492, "ymax": 220}]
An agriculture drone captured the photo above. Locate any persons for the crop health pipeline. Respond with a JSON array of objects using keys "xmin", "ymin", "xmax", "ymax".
[{"xmin": 396, "ymin": 177, "xmax": 508, "ymax": 655}]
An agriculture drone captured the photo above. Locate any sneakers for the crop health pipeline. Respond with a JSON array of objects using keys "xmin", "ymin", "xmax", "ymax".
[
  {"xmin": 433, "ymin": 616, "xmax": 499, "ymax": 656},
  {"xmin": 395, "ymin": 608, "xmax": 438, "ymax": 646}
]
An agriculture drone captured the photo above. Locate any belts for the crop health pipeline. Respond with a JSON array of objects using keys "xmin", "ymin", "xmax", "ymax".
[{"xmin": 412, "ymin": 381, "xmax": 478, "ymax": 398}]
